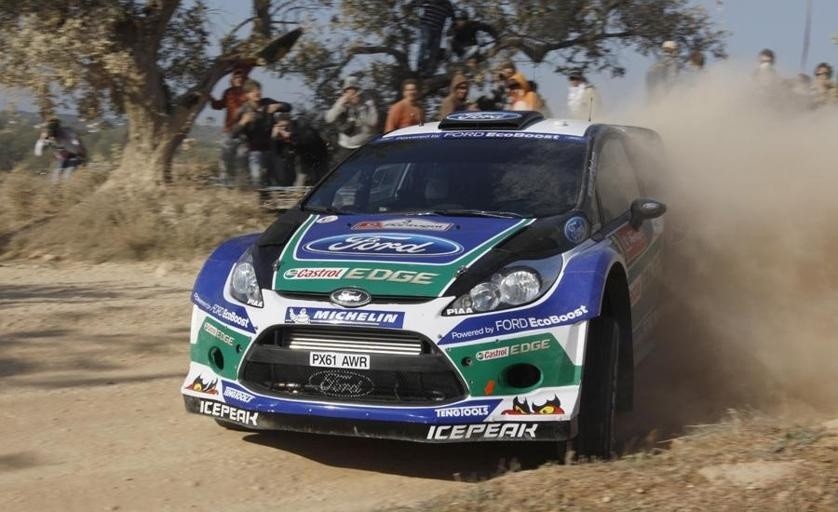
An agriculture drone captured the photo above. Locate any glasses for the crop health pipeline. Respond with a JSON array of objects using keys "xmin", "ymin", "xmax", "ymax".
[
  {"xmin": 457, "ymin": 86, "xmax": 468, "ymax": 89},
  {"xmin": 568, "ymin": 76, "xmax": 579, "ymax": 80}
]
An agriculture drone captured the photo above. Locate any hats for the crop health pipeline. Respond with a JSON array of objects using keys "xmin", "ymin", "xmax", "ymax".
[
  {"xmin": 342, "ymin": 76, "xmax": 361, "ymax": 91},
  {"xmin": 660, "ymin": 40, "xmax": 679, "ymax": 50},
  {"xmin": 504, "ymin": 78, "xmax": 522, "ymax": 89},
  {"xmin": 568, "ymin": 67, "xmax": 585, "ymax": 77}
]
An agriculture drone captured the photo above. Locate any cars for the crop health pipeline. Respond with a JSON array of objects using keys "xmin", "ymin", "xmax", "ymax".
[{"xmin": 182, "ymin": 111, "xmax": 697, "ymax": 460}]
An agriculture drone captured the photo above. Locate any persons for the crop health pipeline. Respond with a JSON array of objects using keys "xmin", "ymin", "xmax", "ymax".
[
  {"xmin": 647, "ymin": 41, "xmax": 838, "ymax": 112},
  {"xmin": 568, "ymin": 67, "xmax": 600, "ymax": 123},
  {"xmin": 445, "ymin": 10, "xmax": 497, "ymax": 59},
  {"xmin": 35, "ymin": 115, "xmax": 85, "ymax": 180}
]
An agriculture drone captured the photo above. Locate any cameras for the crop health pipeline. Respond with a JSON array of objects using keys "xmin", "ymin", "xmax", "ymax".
[
  {"xmin": 47, "ymin": 128, "xmax": 56, "ymax": 138},
  {"xmin": 255, "ymin": 111, "xmax": 264, "ymax": 119}
]
